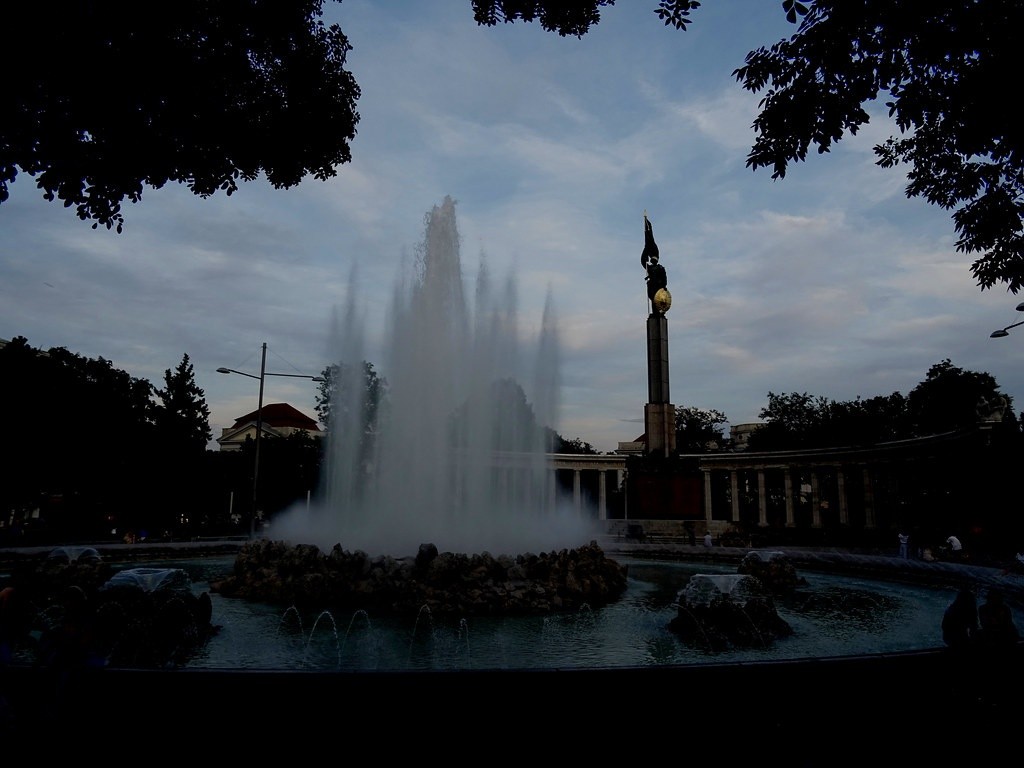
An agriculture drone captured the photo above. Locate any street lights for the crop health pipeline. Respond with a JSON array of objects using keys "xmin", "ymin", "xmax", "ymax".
[{"xmin": 216, "ymin": 341, "xmax": 326, "ymax": 542}]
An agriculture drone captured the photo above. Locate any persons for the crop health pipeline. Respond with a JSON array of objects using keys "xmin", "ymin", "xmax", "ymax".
[
  {"xmin": 0, "ymin": 610, "xmax": 23, "ymax": 758},
  {"xmin": 0, "ymin": 587, "xmax": 16, "ymax": 609},
  {"xmin": 941, "ymin": 583, "xmax": 1024, "ymax": 673},
  {"xmin": 896, "ymin": 527, "xmax": 964, "ymax": 562},
  {"xmin": 124, "ymin": 534, "xmax": 132, "ymax": 544},
  {"xmin": 704, "ymin": 530, "xmax": 713, "ymax": 547}
]
